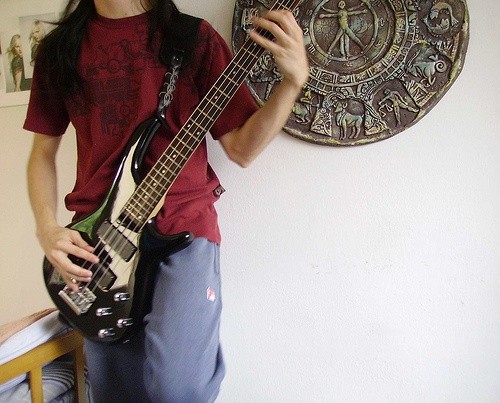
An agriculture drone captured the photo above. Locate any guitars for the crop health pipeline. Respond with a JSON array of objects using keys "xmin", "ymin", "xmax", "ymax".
[{"xmin": 42, "ymin": 0, "xmax": 302, "ymax": 344}]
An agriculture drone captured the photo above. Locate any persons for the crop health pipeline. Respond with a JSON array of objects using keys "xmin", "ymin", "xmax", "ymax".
[
  {"xmin": 5, "ymin": 34, "xmax": 29, "ymax": 91},
  {"xmin": 315, "ymin": 1, "xmax": 371, "ymax": 57},
  {"xmin": 29, "ymin": 21, "xmax": 47, "ymax": 66},
  {"xmin": 23, "ymin": 1, "xmax": 308, "ymax": 402},
  {"xmin": 377, "ymin": 89, "xmax": 419, "ymax": 128},
  {"xmin": 249, "ymin": 66, "xmax": 287, "ymax": 101}
]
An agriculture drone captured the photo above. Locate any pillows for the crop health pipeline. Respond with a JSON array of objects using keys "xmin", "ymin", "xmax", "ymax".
[{"xmin": 0, "ymin": 308, "xmax": 73, "ymax": 395}]
